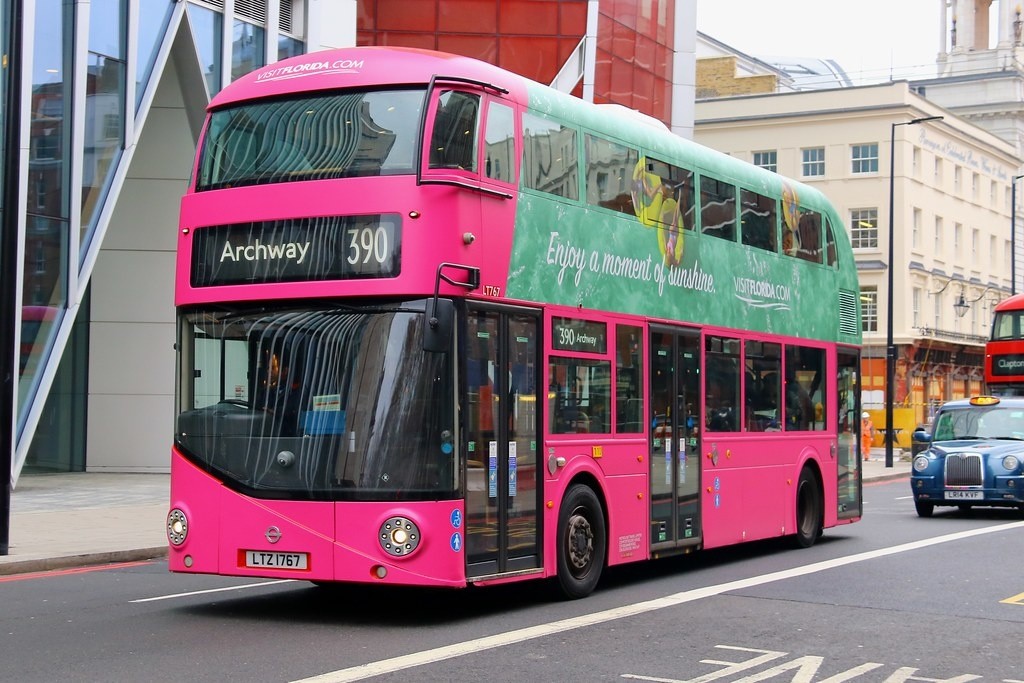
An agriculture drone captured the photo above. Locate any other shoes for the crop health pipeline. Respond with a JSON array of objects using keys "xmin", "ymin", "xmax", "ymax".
[{"xmin": 864, "ymin": 458, "xmax": 868, "ymax": 461}]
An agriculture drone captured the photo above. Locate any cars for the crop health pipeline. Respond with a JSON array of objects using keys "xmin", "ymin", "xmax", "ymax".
[{"xmin": 911, "ymin": 394, "xmax": 1024, "ymax": 520}]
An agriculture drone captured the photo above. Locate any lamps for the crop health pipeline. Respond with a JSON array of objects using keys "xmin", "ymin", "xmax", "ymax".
[
  {"xmin": 968, "ymin": 286, "xmax": 1007, "ymax": 323},
  {"xmin": 928, "ymin": 278, "xmax": 970, "ymax": 317}
]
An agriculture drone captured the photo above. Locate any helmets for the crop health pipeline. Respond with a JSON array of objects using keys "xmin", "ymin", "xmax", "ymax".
[{"xmin": 861, "ymin": 412, "xmax": 870, "ymax": 418}]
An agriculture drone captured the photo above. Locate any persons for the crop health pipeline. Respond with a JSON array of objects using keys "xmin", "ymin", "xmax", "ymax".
[
  {"xmin": 861, "ymin": 412, "xmax": 874, "ymax": 461},
  {"xmin": 258, "ymin": 353, "xmax": 294, "ymax": 436},
  {"xmin": 629, "ymin": 168, "xmax": 681, "ymax": 266}
]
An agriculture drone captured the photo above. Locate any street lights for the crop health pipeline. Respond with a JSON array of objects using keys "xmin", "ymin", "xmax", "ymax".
[{"xmin": 884, "ymin": 115, "xmax": 945, "ymax": 468}]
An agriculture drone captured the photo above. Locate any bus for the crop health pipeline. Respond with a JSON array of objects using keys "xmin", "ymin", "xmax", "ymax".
[
  {"xmin": 983, "ymin": 293, "xmax": 1024, "ymax": 398},
  {"xmin": 165, "ymin": 45, "xmax": 864, "ymax": 602}
]
